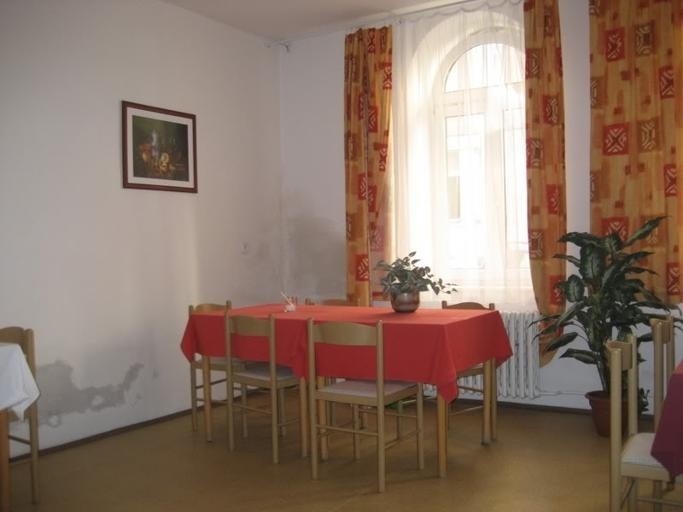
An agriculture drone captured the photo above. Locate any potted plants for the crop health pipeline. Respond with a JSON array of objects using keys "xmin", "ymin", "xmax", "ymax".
[{"xmin": 524, "ymin": 216, "xmax": 683, "ymax": 438}]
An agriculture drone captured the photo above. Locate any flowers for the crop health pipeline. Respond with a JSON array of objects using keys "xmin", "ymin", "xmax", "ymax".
[{"xmin": 375, "ymin": 250, "xmax": 458, "ymax": 299}]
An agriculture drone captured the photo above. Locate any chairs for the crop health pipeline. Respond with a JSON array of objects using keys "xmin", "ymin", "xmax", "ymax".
[
  {"xmin": 305, "ymin": 318, "xmax": 427, "ymax": 492},
  {"xmin": 224, "ymin": 310, "xmax": 326, "ymax": 466},
  {"xmin": 0, "ymin": 325, "xmax": 43, "ymax": 505},
  {"xmin": 188, "ymin": 300, "xmax": 287, "ymax": 443},
  {"xmin": 606, "ymin": 335, "xmax": 683, "ymax": 512},
  {"xmin": 647, "ymin": 313, "xmax": 678, "ymax": 512},
  {"xmin": 396, "ymin": 300, "xmax": 501, "ymax": 442},
  {"xmin": 298, "ymin": 300, "xmax": 370, "ymax": 429}
]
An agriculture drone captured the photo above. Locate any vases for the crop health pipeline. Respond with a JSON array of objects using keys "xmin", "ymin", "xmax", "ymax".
[{"xmin": 390, "ymin": 291, "xmax": 419, "ymax": 313}]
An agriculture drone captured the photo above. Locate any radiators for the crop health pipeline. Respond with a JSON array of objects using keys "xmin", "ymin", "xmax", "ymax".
[{"xmin": 424, "ymin": 310, "xmax": 541, "ymax": 400}]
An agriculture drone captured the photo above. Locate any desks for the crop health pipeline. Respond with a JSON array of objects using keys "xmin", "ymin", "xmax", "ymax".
[
  {"xmin": 650, "ymin": 360, "xmax": 683, "ymax": 477},
  {"xmin": 0, "ymin": 342, "xmax": 40, "ymax": 420},
  {"xmin": 182, "ymin": 304, "xmax": 513, "ymax": 478}
]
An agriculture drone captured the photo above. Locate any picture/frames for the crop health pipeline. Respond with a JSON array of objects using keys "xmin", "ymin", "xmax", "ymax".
[{"xmin": 122, "ymin": 100, "xmax": 200, "ymax": 193}]
